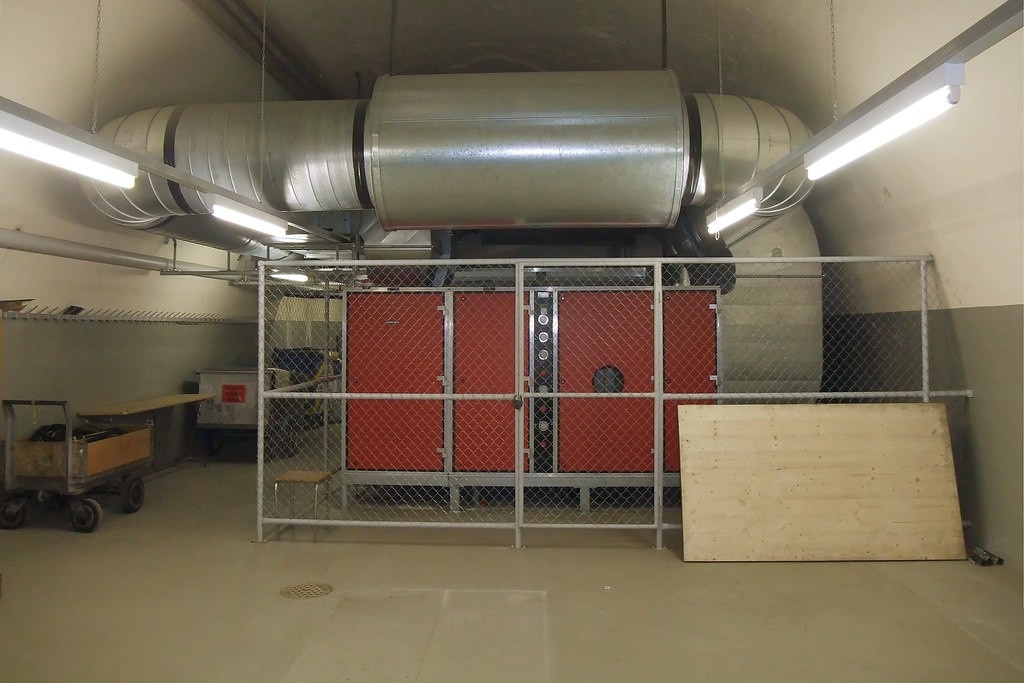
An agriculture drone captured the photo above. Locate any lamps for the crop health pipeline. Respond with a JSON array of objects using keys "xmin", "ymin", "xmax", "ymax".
[
  {"xmin": 204, "ymin": 193, "xmax": 290, "ymax": 239},
  {"xmin": 706, "ymin": 185, "xmax": 765, "ymax": 235},
  {"xmin": 0, "ymin": 97, "xmax": 140, "ymax": 191},
  {"xmin": 801, "ymin": 61, "xmax": 965, "ymax": 185}
]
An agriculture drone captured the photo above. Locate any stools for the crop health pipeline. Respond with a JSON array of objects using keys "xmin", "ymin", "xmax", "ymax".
[{"xmin": 273, "ymin": 470, "xmax": 334, "ymax": 543}]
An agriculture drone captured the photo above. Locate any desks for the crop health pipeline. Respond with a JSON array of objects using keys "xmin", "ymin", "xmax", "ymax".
[{"xmin": 78, "ymin": 392, "xmax": 219, "ymax": 479}]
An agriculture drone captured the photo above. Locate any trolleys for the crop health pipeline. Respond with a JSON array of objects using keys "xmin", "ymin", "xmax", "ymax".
[
  {"xmin": 0, "ymin": 400, "xmax": 154, "ymax": 533},
  {"xmin": 195, "ymin": 367, "xmax": 316, "ymax": 457}
]
